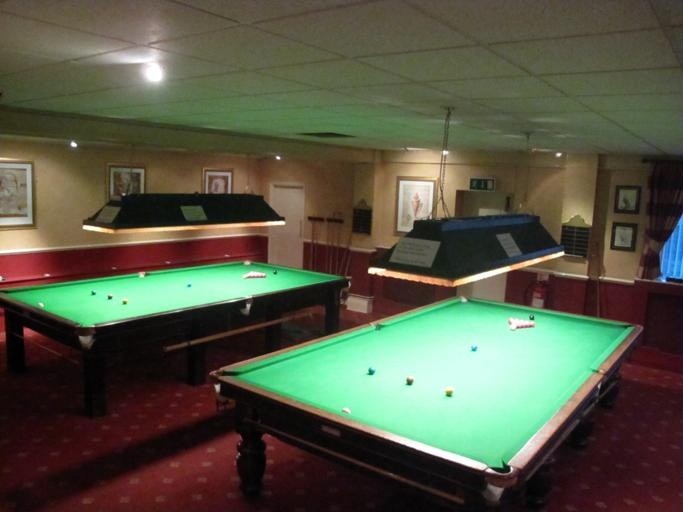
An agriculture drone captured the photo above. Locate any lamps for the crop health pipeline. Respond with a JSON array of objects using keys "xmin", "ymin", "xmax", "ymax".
[
  {"xmin": 367, "ymin": 106, "xmax": 566, "ymax": 288},
  {"xmin": 82, "ymin": 144, "xmax": 286, "ymax": 235}
]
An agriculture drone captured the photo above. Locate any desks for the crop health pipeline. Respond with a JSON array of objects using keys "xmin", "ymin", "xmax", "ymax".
[
  {"xmin": 210, "ymin": 293, "xmax": 645, "ymax": 512},
  {"xmin": 0, "ymin": 260, "xmax": 348, "ymax": 418}
]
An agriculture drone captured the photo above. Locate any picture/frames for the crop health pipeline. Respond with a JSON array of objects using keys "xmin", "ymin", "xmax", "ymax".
[
  {"xmin": 611, "ymin": 223, "xmax": 638, "ymax": 251},
  {"xmin": 0, "ymin": 158, "xmax": 37, "ymax": 230},
  {"xmin": 394, "ymin": 176, "xmax": 438, "ymax": 236},
  {"xmin": 614, "ymin": 185, "xmax": 640, "ymax": 214},
  {"xmin": 203, "ymin": 168, "xmax": 234, "ymax": 194},
  {"xmin": 106, "ymin": 163, "xmax": 146, "ymax": 203}
]
